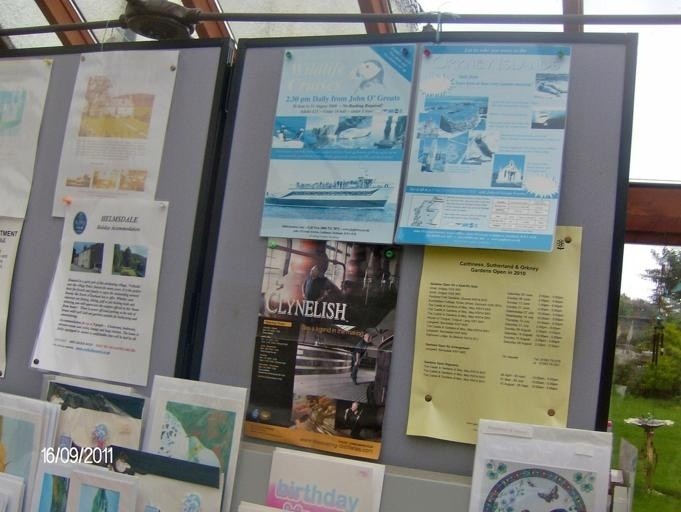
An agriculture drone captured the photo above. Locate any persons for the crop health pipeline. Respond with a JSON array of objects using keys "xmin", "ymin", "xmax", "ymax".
[{"xmin": 289, "ymin": 266, "xmax": 396, "ymax": 447}]
